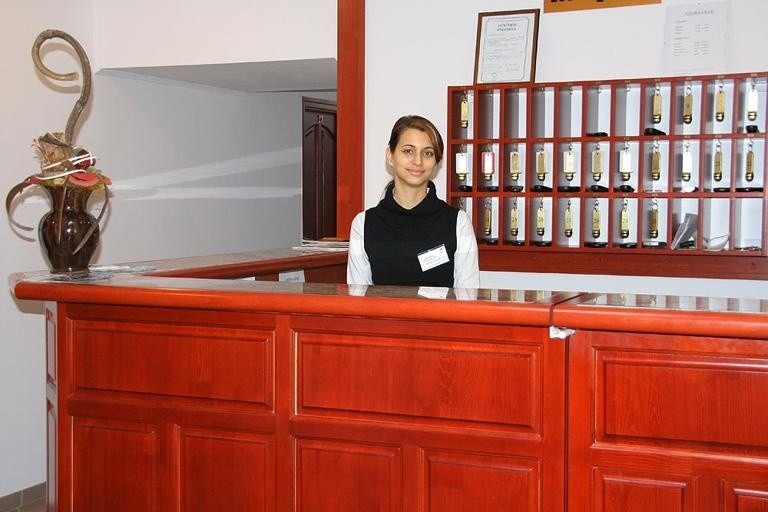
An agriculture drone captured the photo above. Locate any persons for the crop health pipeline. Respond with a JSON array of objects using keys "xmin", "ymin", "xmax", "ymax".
[{"xmin": 347, "ymin": 115, "xmax": 479, "ymax": 288}]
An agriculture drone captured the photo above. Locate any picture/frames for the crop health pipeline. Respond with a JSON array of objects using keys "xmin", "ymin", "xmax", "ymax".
[{"xmin": 472, "ymin": 8, "xmax": 539, "ymax": 85}]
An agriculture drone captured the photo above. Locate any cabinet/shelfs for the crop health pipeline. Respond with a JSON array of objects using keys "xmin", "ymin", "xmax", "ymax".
[{"xmin": 447, "ymin": 71, "xmax": 768, "ymax": 283}]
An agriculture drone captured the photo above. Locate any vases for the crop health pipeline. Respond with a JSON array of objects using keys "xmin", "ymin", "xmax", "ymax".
[{"xmin": 38, "ymin": 186, "xmax": 100, "ymax": 273}]
